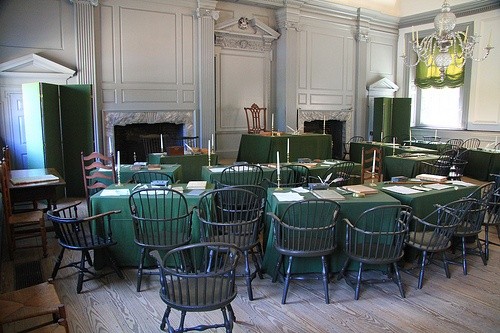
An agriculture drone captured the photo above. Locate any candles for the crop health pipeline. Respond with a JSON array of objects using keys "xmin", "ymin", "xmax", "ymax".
[
  {"xmin": 287, "ymin": 138, "xmax": 289, "ymax": 158},
  {"xmin": 117, "ymin": 151, "xmax": 120, "ymax": 172},
  {"xmin": 276, "ymin": 151, "xmax": 280, "ymax": 175}
]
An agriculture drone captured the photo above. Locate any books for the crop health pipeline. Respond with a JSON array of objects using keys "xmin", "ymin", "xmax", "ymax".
[
  {"xmin": 131, "ymin": 162, "xmax": 161, "ymax": 171},
  {"xmin": 311, "ymin": 189, "xmax": 346, "ymax": 201},
  {"xmin": 187, "ymin": 180, "xmax": 208, "ymax": 190},
  {"xmin": 416, "ymin": 173, "xmax": 447, "ymax": 183},
  {"xmin": 343, "ymin": 185, "xmax": 378, "ymax": 194}
]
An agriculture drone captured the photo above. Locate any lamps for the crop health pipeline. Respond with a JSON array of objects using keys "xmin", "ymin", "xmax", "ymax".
[{"xmin": 398, "ymin": 0, "xmax": 495, "ymax": 82}]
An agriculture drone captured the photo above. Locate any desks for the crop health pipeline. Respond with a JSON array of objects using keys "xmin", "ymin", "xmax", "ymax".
[
  {"xmin": 260, "ymin": 185, "xmax": 401, "ymax": 281},
  {"xmin": 236, "ymin": 134, "xmax": 333, "ymax": 165},
  {"xmin": 285, "ymin": 159, "xmax": 362, "ymax": 187},
  {"xmin": 349, "ymin": 141, "xmax": 400, "ymax": 173},
  {"xmin": 89, "ymin": 164, "xmax": 183, "ymax": 193},
  {"xmin": 366, "ymin": 177, "xmax": 481, "ymax": 265},
  {"xmin": 146, "ymin": 153, "xmax": 218, "ymax": 183},
  {"xmin": 383, "ymin": 153, "xmax": 450, "ymax": 182},
  {"xmin": 453, "ymin": 146, "xmax": 500, "ymax": 183},
  {"xmin": 201, "ymin": 166, "xmax": 294, "ymax": 205},
  {"xmin": 90, "ymin": 184, "xmax": 217, "ymax": 270},
  {"xmin": 402, "ymin": 140, "xmax": 455, "ymax": 156},
  {"xmin": 8, "ymin": 168, "xmax": 67, "ymax": 239},
  {"xmin": 381, "ymin": 146, "xmax": 439, "ymax": 174}
]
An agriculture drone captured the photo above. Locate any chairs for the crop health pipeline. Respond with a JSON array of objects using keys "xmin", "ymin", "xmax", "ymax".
[
  {"xmin": 0, "ymin": 138, "xmax": 500, "ymax": 333},
  {"xmin": 244, "ymin": 103, "xmax": 284, "ymax": 137}
]
